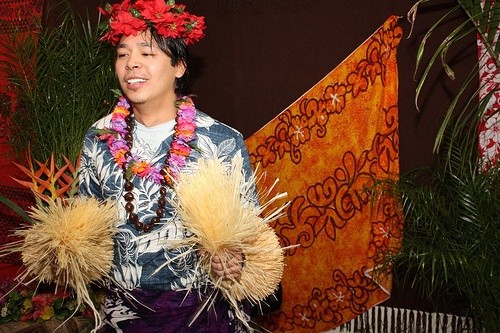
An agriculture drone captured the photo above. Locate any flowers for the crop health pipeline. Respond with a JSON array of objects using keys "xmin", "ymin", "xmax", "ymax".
[{"xmin": 96, "ymin": 0, "xmax": 208, "ymax": 49}]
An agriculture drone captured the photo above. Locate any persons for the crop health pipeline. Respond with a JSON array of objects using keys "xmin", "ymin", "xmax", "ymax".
[{"xmin": 64, "ymin": 0, "xmax": 267, "ymax": 333}]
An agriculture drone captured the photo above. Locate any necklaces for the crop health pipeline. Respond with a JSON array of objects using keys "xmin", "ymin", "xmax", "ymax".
[
  {"xmin": 99, "ymin": 92, "xmax": 198, "ymax": 186},
  {"xmin": 121, "ymin": 95, "xmax": 181, "ymax": 232}
]
